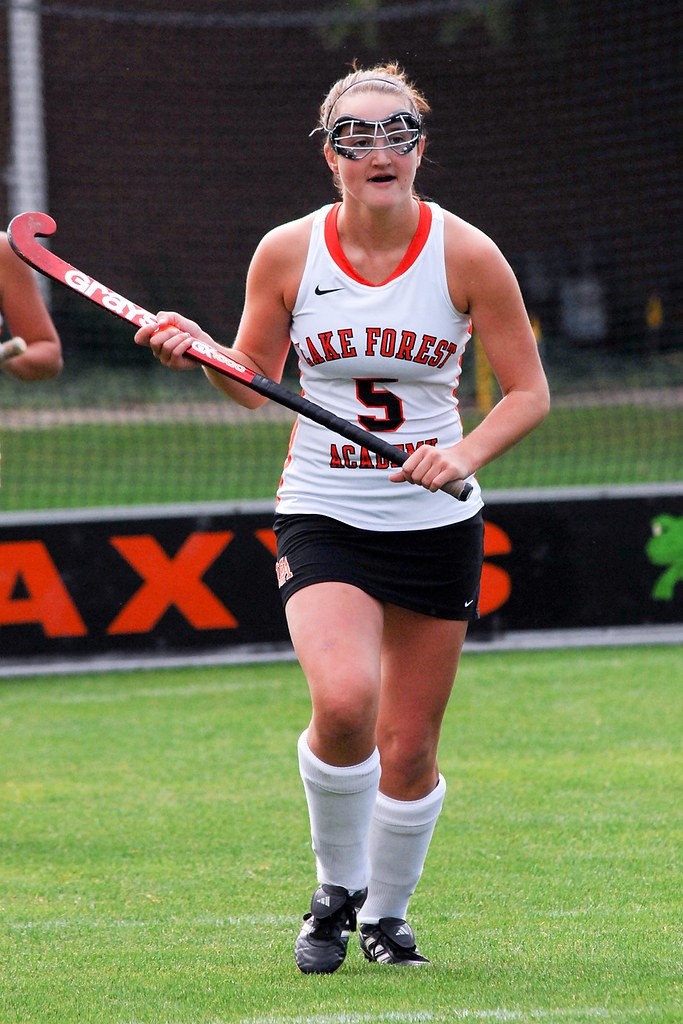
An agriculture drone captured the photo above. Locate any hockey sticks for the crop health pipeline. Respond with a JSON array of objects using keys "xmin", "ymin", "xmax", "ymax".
[{"xmin": 7, "ymin": 210, "xmax": 475, "ymax": 504}]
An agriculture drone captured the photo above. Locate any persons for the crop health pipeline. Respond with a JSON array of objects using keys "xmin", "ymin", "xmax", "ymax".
[
  {"xmin": 134, "ymin": 60, "xmax": 553, "ymax": 973},
  {"xmin": 0, "ymin": 229, "xmax": 64, "ymax": 382}
]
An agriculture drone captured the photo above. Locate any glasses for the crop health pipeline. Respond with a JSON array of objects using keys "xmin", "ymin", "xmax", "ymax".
[{"xmin": 326, "ymin": 110, "xmax": 422, "ymax": 161}]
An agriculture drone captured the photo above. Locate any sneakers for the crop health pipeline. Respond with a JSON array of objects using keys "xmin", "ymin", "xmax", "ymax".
[
  {"xmin": 359, "ymin": 916, "xmax": 429, "ymax": 965},
  {"xmin": 294, "ymin": 883, "xmax": 368, "ymax": 975}
]
